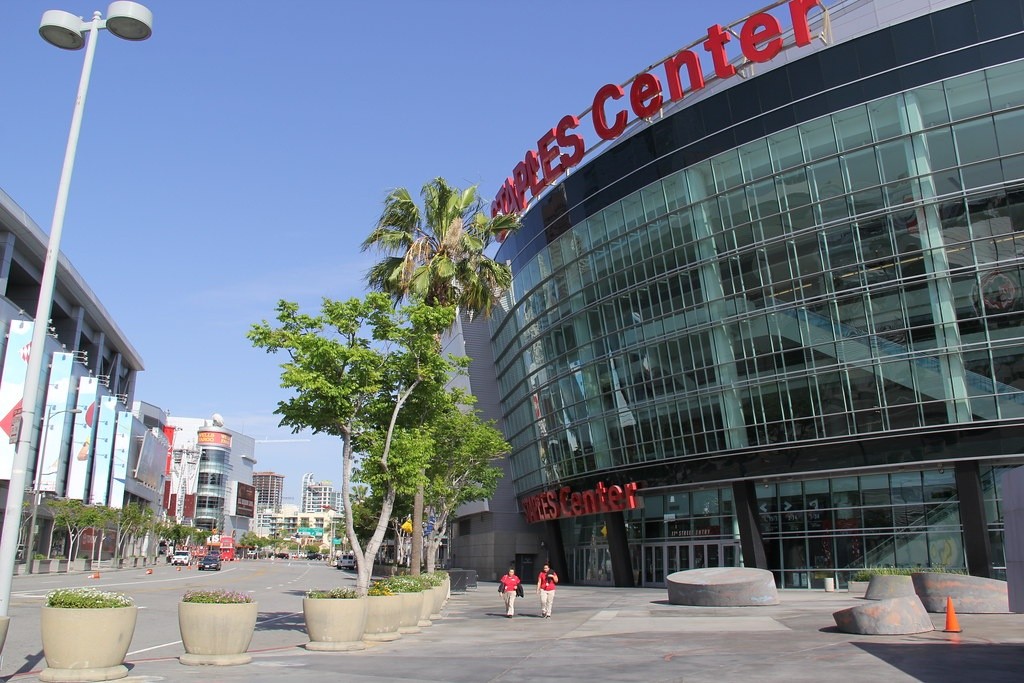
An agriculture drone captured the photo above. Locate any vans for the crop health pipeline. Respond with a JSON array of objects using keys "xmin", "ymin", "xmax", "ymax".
[{"xmin": 337, "ymin": 553, "xmax": 355, "ymax": 570}]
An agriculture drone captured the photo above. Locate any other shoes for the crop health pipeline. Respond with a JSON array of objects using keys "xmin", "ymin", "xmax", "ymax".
[
  {"xmin": 542, "ymin": 614, "xmax": 546, "ymax": 617},
  {"xmin": 508, "ymin": 615, "xmax": 512, "ymax": 618},
  {"xmin": 547, "ymin": 615, "xmax": 550, "ymax": 617}
]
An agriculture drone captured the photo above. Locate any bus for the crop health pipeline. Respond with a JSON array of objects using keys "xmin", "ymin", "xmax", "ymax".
[{"xmin": 220, "ymin": 547, "xmax": 234, "ymax": 561}]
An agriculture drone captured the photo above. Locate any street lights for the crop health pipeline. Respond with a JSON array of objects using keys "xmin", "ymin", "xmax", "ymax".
[
  {"xmin": 16, "ymin": 544, "xmax": 28, "ymax": 559},
  {"xmin": 24, "ymin": 408, "xmax": 82, "ymax": 576},
  {"xmin": 210, "ymin": 506, "xmax": 225, "ymax": 550},
  {"xmin": 0, "ymin": 1, "xmax": 156, "ymax": 653}
]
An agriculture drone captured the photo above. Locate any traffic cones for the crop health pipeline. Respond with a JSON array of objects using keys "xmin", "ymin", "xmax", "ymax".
[
  {"xmin": 942, "ymin": 597, "xmax": 963, "ymax": 634},
  {"xmin": 146, "ymin": 569, "xmax": 152, "ymax": 575},
  {"xmin": 187, "ymin": 561, "xmax": 191, "ymax": 570},
  {"xmin": 86, "ymin": 571, "xmax": 100, "ymax": 579},
  {"xmin": 176, "ymin": 567, "xmax": 181, "ymax": 571}
]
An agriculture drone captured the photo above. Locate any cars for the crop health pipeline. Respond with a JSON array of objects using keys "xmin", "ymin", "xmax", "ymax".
[
  {"xmin": 307, "ymin": 552, "xmax": 322, "ymax": 560},
  {"xmin": 333, "ymin": 558, "xmax": 338, "ymax": 567},
  {"xmin": 208, "ymin": 551, "xmax": 220, "ymax": 558},
  {"xmin": 274, "ymin": 553, "xmax": 289, "ymax": 559},
  {"xmin": 198, "ymin": 555, "xmax": 222, "ymax": 571}
]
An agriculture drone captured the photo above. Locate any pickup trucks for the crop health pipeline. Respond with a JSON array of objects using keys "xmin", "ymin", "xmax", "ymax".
[{"xmin": 171, "ymin": 551, "xmax": 193, "ymax": 565}]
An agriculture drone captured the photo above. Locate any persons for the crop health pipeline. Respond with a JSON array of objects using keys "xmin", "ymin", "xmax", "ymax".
[
  {"xmin": 536, "ymin": 564, "xmax": 558, "ymax": 619},
  {"xmin": 500, "ymin": 567, "xmax": 520, "ymax": 618}
]
